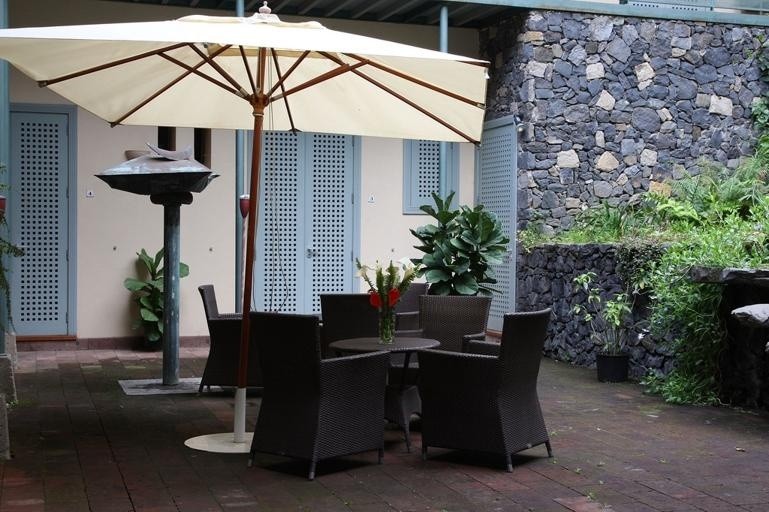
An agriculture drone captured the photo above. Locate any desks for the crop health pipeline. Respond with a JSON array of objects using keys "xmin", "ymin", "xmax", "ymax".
[{"xmin": 329, "ymin": 337, "xmax": 440, "ymax": 453}]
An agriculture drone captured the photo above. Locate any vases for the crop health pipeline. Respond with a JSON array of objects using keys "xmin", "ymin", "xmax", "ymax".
[{"xmin": 378, "ymin": 307, "xmax": 396, "ymax": 344}]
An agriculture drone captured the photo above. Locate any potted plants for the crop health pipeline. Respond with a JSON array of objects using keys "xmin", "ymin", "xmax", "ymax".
[
  {"xmin": 568, "ymin": 272, "xmax": 641, "ymax": 382},
  {"xmin": 124, "ymin": 248, "xmax": 189, "ymax": 352}
]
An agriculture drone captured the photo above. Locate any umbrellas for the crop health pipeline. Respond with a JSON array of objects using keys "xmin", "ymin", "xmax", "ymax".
[{"xmin": 0, "ymin": 1, "xmax": 490, "ymax": 444}]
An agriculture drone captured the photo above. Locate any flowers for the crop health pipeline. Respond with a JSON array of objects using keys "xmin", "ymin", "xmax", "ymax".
[{"xmin": 355, "ymin": 257, "xmax": 422, "ymax": 343}]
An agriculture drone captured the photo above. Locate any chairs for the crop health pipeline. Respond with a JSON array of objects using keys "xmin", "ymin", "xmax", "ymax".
[
  {"xmin": 319, "ymin": 294, "xmax": 379, "ymax": 359},
  {"xmin": 394, "ymin": 295, "xmax": 492, "ymax": 370},
  {"xmin": 199, "ymin": 285, "xmax": 264, "ymax": 392},
  {"xmin": 416, "ymin": 308, "xmax": 553, "ymax": 473},
  {"xmin": 247, "ymin": 312, "xmax": 391, "ymax": 481},
  {"xmin": 396, "ymin": 283, "xmax": 429, "ymax": 330}
]
